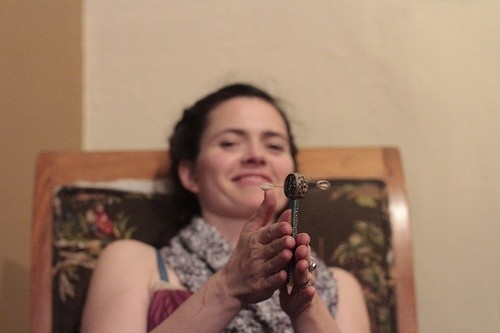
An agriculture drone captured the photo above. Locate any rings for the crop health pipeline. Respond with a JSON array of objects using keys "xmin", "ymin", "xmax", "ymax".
[
  {"xmin": 305, "ymin": 270, "xmax": 312, "ymax": 289},
  {"xmin": 309, "ymin": 257, "xmax": 317, "ymax": 270}
]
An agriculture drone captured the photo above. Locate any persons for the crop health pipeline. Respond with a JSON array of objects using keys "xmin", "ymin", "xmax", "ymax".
[{"xmin": 80, "ymin": 83, "xmax": 370, "ymax": 333}]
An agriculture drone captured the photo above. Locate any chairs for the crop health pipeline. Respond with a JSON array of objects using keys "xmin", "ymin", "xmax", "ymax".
[{"xmin": 33, "ymin": 145, "xmax": 418, "ymax": 333}]
